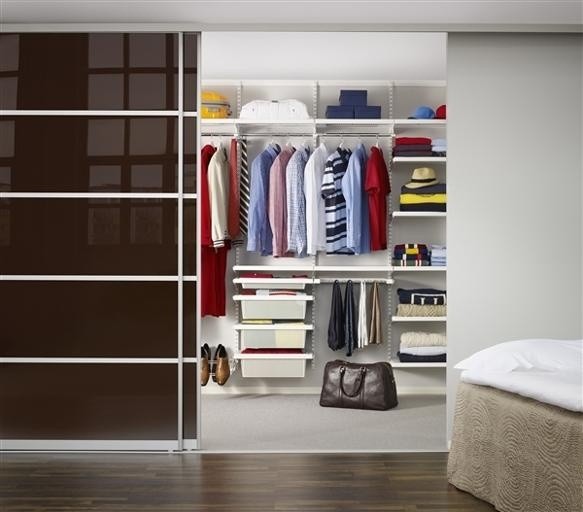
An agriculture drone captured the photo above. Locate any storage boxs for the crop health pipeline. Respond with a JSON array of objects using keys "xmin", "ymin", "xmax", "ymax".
[{"xmin": 233, "ymin": 294, "xmax": 314, "ymax": 378}]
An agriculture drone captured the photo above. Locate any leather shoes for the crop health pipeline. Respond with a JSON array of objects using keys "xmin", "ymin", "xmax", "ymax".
[
  {"xmin": 200, "ymin": 343, "xmax": 210, "ymax": 386},
  {"xmin": 213, "ymin": 344, "xmax": 230, "ymax": 386}
]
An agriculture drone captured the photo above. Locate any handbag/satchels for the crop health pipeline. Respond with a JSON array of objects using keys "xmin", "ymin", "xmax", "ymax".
[
  {"xmin": 316, "ymin": 359, "xmax": 400, "ymax": 414},
  {"xmin": 239, "ymin": 99, "xmax": 310, "ymax": 120}
]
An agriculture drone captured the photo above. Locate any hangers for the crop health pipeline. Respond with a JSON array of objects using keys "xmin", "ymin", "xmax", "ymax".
[{"xmin": 201, "ymin": 128, "xmax": 382, "ymax": 151}]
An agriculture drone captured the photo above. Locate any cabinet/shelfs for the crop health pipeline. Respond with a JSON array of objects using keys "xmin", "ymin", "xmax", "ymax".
[{"xmin": 199, "ymin": 80, "xmax": 449, "ymax": 394}]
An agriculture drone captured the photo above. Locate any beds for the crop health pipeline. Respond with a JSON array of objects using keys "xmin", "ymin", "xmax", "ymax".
[{"xmin": 444, "ymin": 370, "xmax": 583, "ymax": 512}]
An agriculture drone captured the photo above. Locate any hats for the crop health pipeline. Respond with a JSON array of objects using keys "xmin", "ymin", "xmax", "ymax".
[
  {"xmin": 433, "ymin": 105, "xmax": 446, "ymax": 119},
  {"xmin": 408, "ymin": 106, "xmax": 435, "ymax": 119},
  {"xmin": 405, "ymin": 167, "xmax": 440, "ymax": 190}
]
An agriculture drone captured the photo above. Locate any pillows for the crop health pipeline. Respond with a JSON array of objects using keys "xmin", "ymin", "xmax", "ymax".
[{"xmin": 453, "ymin": 339, "xmax": 583, "ymax": 375}]
